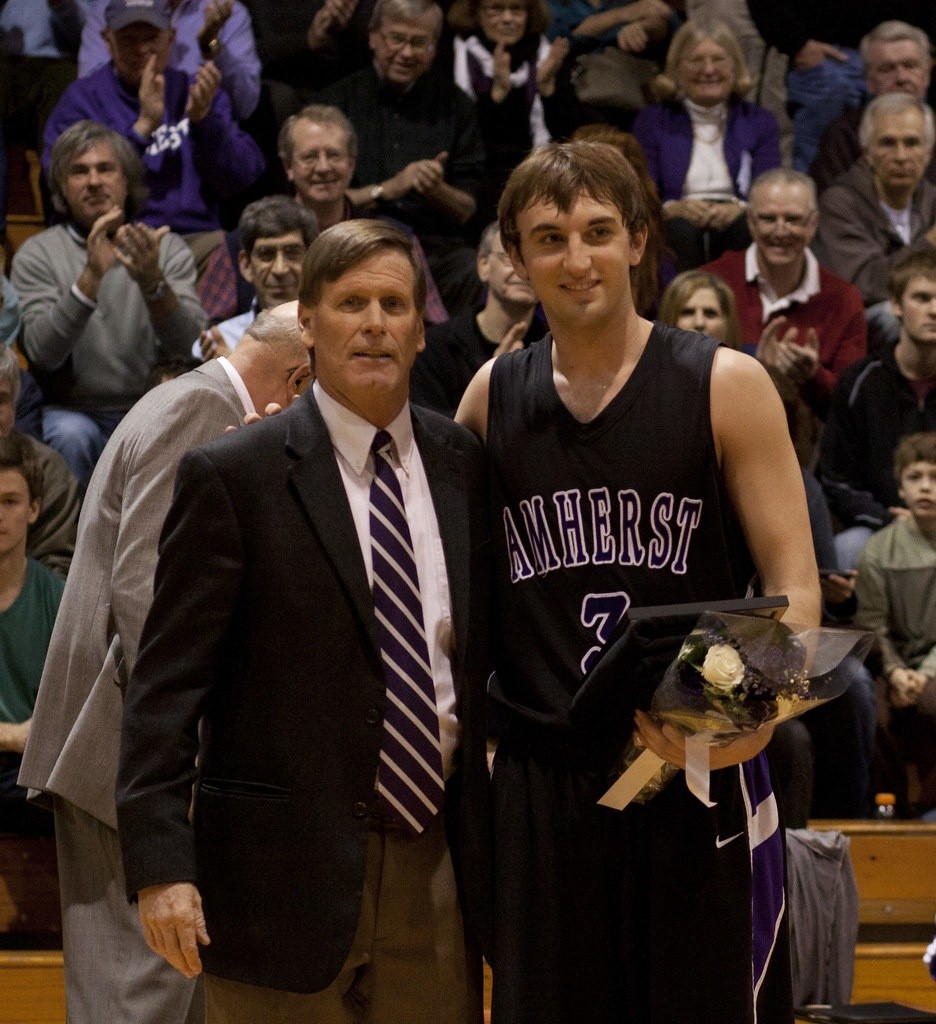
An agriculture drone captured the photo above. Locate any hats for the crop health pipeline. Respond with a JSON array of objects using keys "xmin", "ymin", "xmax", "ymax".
[{"xmin": 103, "ymin": 0, "xmax": 171, "ymax": 31}]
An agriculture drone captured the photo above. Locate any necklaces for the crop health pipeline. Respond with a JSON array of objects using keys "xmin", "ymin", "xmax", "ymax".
[{"xmin": 695, "ymin": 119, "xmax": 723, "ymax": 143}]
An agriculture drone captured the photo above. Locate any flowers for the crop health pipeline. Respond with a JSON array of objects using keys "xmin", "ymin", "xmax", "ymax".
[{"xmin": 675, "ymin": 626, "xmax": 816, "ymax": 737}]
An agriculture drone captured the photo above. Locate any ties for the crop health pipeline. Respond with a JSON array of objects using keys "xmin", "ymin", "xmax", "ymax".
[{"xmin": 371, "ymin": 429, "xmax": 447, "ymax": 836}]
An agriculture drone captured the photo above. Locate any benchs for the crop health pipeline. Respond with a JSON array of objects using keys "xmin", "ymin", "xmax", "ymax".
[
  {"xmin": 5, "ymin": 151, "xmax": 47, "ymax": 253},
  {"xmin": 0, "ymin": 824, "xmax": 936, "ymax": 1024}
]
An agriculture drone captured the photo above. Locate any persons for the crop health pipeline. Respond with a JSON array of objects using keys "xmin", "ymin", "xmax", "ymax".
[
  {"xmin": 1, "ymin": 0, "xmax": 936, "ymax": 934},
  {"xmin": 223, "ymin": 139, "xmax": 821, "ymax": 1024},
  {"xmin": 115, "ymin": 219, "xmax": 486, "ymax": 1024}
]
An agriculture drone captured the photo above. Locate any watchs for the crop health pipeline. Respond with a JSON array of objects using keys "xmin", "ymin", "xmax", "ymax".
[
  {"xmin": 370, "ymin": 182, "xmax": 387, "ymax": 204},
  {"xmin": 144, "ymin": 280, "xmax": 169, "ymax": 302},
  {"xmin": 201, "ymin": 38, "xmax": 220, "ymax": 57}
]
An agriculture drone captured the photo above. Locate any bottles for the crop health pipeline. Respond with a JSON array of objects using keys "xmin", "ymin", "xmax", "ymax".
[{"xmin": 875, "ymin": 792, "xmax": 896, "ymax": 820}]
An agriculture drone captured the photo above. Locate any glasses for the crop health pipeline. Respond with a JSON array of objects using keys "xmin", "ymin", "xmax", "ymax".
[
  {"xmin": 379, "ymin": 29, "xmax": 434, "ymax": 48},
  {"xmin": 748, "ymin": 208, "xmax": 818, "ymax": 236},
  {"xmin": 489, "ymin": 249, "xmax": 513, "ymax": 265},
  {"xmin": 254, "ymin": 244, "xmax": 306, "ymax": 261},
  {"xmin": 483, "ymin": 3, "xmax": 528, "ymax": 18}
]
